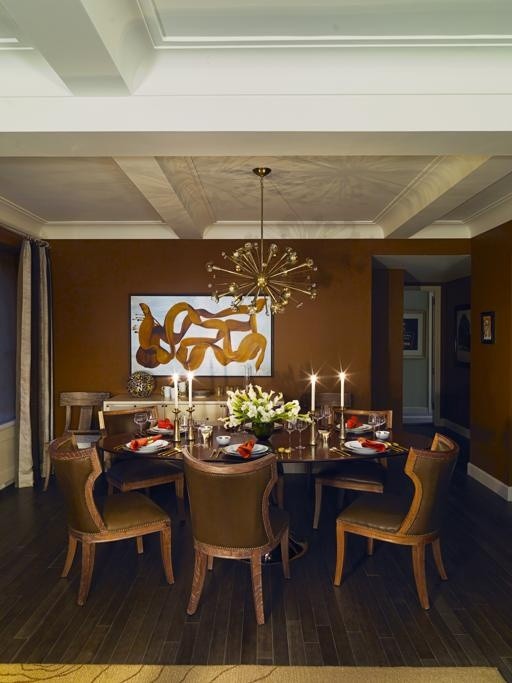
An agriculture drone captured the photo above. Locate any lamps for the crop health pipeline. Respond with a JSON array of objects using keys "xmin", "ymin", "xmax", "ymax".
[{"xmin": 205, "ymin": 167, "xmax": 318, "ymax": 314}]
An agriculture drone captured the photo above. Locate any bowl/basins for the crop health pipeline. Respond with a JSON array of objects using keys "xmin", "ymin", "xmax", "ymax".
[
  {"xmin": 375, "ymin": 430, "xmax": 390, "ymax": 439},
  {"xmin": 192, "ymin": 389, "xmax": 211, "ymax": 398},
  {"xmin": 216, "ymin": 435, "xmax": 231, "ymax": 445}
]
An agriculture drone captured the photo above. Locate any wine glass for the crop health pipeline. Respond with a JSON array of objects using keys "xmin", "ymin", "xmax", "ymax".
[
  {"xmin": 134, "ymin": 405, "xmax": 158, "ymax": 435},
  {"xmin": 283, "ymin": 403, "xmax": 333, "ymax": 449},
  {"xmin": 367, "ymin": 412, "xmax": 386, "ymax": 435}
]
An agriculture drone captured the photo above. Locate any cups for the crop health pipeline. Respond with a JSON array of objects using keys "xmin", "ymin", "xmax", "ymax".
[
  {"xmin": 197, "ymin": 425, "xmax": 213, "ymax": 445},
  {"xmin": 179, "ymin": 380, "xmax": 186, "ymax": 397},
  {"xmin": 214, "ymin": 385, "xmax": 242, "ymax": 398},
  {"xmin": 163, "ymin": 385, "xmax": 171, "ymax": 398}
]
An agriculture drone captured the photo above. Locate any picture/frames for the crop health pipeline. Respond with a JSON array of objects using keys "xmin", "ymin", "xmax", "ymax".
[
  {"xmin": 480, "ymin": 311, "xmax": 494, "ymax": 342},
  {"xmin": 402, "ymin": 310, "xmax": 425, "ymax": 358},
  {"xmin": 128, "ymin": 292, "xmax": 273, "ymax": 377}
]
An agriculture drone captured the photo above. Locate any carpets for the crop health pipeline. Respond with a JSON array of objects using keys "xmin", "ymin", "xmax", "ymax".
[{"xmin": 0, "ymin": 662, "xmax": 507, "ymax": 683}]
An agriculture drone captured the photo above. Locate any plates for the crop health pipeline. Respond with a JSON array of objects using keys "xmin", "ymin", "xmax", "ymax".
[
  {"xmin": 343, "ymin": 440, "xmax": 389, "ymax": 454},
  {"xmin": 221, "ymin": 444, "xmax": 269, "ymax": 457},
  {"xmin": 148, "ymin": 426, "xmax": 183, "ymax": 434},
  {"xmin": 124, "ymin": 439, "xmax": 169, "ymax": 453},
  {"xmin": 335, "ymin": 421, "xmax": 371, "ymax": 433}
]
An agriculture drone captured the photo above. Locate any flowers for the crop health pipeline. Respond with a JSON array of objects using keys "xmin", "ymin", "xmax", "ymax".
[{"xmin": 220, "ymin": 383, "xmax": 310, "ymax": 428}]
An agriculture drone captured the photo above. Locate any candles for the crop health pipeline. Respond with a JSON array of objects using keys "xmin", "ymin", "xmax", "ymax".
[
  {"xmin": 340, "ymin": 374, "xmax": 345, "ymax": 406},
  {"xmin": 310, "ymin": 375, "xmax": 314, "ymax": 411},
  {"xmin": 189, "ymin": 377, "xmax": 193, "ymax": 408},
  {"xmin": 174, "ymin": 378, "xmax": 179, "ymax": 407}
]
